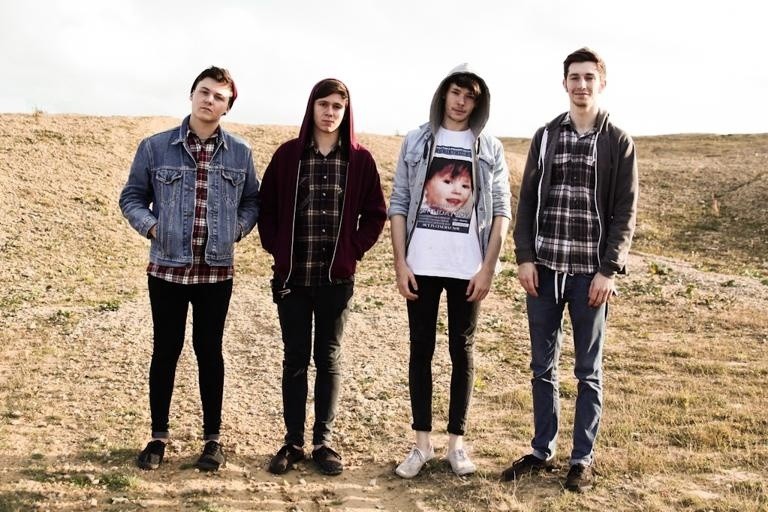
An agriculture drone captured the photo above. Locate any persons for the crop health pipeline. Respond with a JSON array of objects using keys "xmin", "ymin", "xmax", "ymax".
[
  {"xmin": 499, "ymin": 46, "xmax": 640, "ymax": 491},
  {"xmin": 389, "ymin": 62, "xmax": 514, "ymax": 478},
  {"xmin": 421, "ymin": 157, "xmax": 474, "ymax": 216},
  {"xmin": 258, "ymin": 78, "xmax": 388, "ymax": 476},
  {"xmin": 120, "ymin": 66, "xmax": 259, "ymax": 470}
]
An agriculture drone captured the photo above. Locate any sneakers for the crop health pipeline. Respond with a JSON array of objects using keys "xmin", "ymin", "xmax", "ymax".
[
  {"xmin": 395, "ymin": 445, "xmax": 435, "ymax": 478},
  {"xmin": 197, "ymin": 440, "xmax": 224, "ymax": 469},
  {"xmin": 312, "ymin": 445, "xmax": 343, "ymax": 475},
  {"xmin": 139, "ymin": 440, "xmax": 166, "ymax": 469},
  {"xmin": 502, "ymin": 453, "xmax": 548, "ymax": 481},
  {"xmin": 448, "ymin": 448, "xmax": 475, "ymax": 475},
  {"xmin": 269, "ymin": 444, "xmax": 304, "ymax": 473},
  {"xmin": 565, "ymin": 461, "xmax": 592, "ymax": 490}
]
